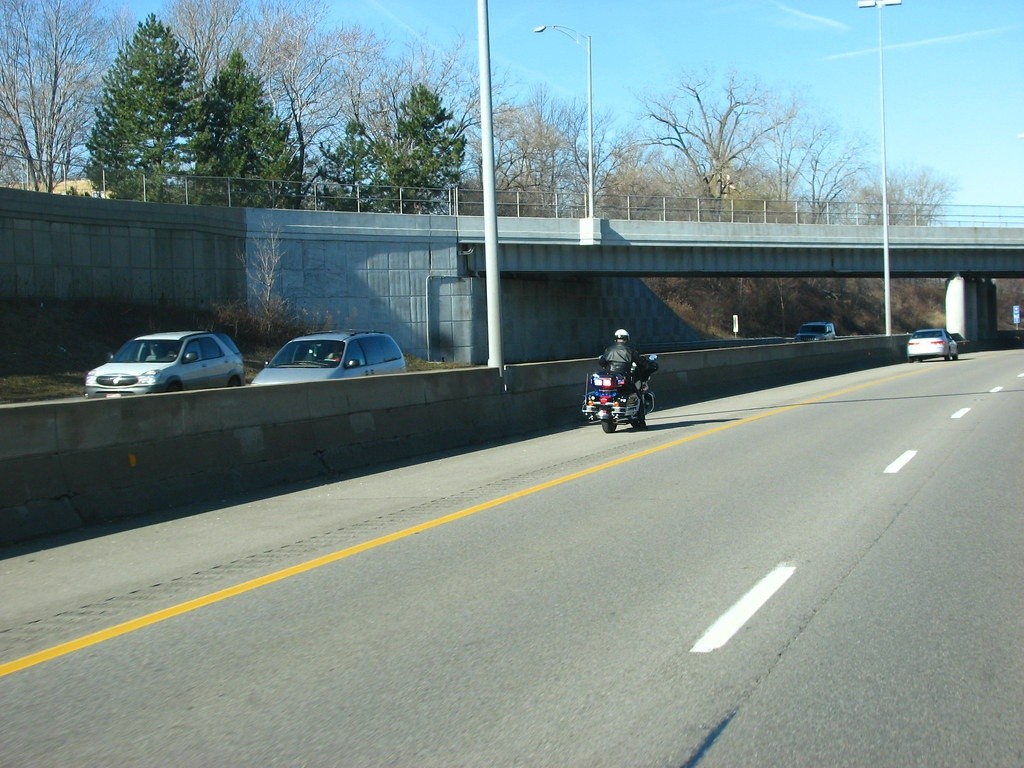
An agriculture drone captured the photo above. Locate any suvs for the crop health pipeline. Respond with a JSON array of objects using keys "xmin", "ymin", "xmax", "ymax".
[
  {"xmin": 252, "ymin": 331, "xmax": 407, "ymax": 385},
  {"xmin": 793, "ymin": 321, "xmax": 835, "ymax": 342}
]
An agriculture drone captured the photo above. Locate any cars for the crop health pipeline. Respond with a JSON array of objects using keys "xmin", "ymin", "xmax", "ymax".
[
  {"xmin": 83, "ymin": 332, "xmax": 246, "ymax": 399},
  {"xmin": 906, "ymin": 329, "xmax": 958, "ymax": 363}
]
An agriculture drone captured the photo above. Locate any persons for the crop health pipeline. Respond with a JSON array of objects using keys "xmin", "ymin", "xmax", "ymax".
[{"xmin": 599, "ymin": 328, "xmax": 655, "ymax": 420}]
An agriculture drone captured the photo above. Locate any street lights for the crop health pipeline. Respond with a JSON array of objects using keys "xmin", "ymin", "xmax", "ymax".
[
  {"xmin": 857, "ymin": 0, "xmax": 902, "ymax": 337},
  {"xmin": 535, "ymin": 24, "xmax": 594, "ymax": 217}
]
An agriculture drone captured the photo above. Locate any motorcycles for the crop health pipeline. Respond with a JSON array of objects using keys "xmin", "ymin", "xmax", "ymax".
[{"xmin": 581, "ymin": 354, "xmax": 658, "ymax": 433}]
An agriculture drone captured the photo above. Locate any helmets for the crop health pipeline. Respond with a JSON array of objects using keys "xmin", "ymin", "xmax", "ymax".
[{"xmin": 615, "ymin": 329, "xmax": 630, "ymax": 340}]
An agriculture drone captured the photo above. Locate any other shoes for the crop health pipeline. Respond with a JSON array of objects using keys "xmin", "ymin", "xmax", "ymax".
[{"xmin": 633, "ymin": 420, "xmax": 648, "ymax": 430}]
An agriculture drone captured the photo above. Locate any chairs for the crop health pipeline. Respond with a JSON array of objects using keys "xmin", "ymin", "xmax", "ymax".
[
  {"xmin": 145, "ymin": 342, "xmax": 161, "ymax": 361},
  {"xmin": 298, "ymin": 344, "xmax": 318, "ymax": 362}
]
